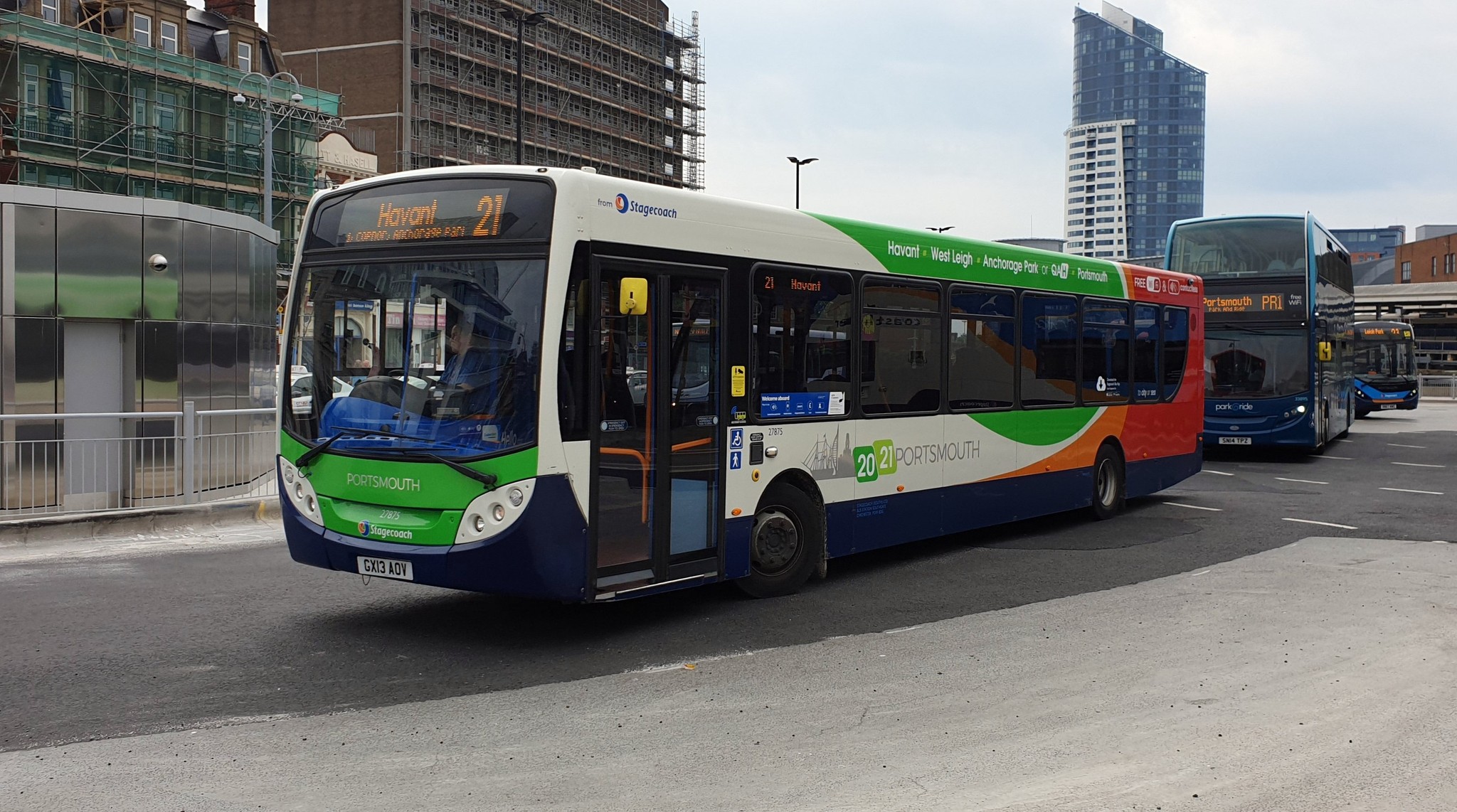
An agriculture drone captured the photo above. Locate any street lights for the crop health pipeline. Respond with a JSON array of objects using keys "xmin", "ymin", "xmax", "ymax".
[
  {"xmin": 492, "ymin": 7, "xmax": 556, "ymax": 166},
  {"xmin": 786, "ymin": 156, "xmax": 821, "ymax": 210},
  {"xmin": 925, "ymin": 226, "xmax": 956, "ymax": 234},
  {"xmin": 232, "ymin": 72, "xmax": 306, "ymax": 231}
]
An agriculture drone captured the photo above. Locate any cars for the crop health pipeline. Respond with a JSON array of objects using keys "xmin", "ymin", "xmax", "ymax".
[
  {"xmin": 274, "ymin": 365, "xmax": 354, "ymax": 415},
  {"xmin": 627, "ymin": 366, "xmax": 648, "ymax": 405},
  {"xmin": 396, "ymin": 362, "xmax": 445, "ymax": 391}
]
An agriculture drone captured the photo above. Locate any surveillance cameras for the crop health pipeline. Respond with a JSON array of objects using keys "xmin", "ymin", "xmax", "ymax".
[
  {"xmin": 233, "ymin": 94, "xmax": 246, "ymax": 107},
  {"xmin": 292, "ymin": 94, "xmax": 303, "ymax": 103}
]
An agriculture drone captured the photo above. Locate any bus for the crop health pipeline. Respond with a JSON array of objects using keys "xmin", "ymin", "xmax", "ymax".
[
  {"xmin": 1354, "ymin": 320, "xmax": 1420, "ymax": 417},
  {"xmin": 672, "ymin": 322, "xmax": 846, "ymax": 405},
  {"xmin": 272, "ymin": 164, "xmax": 1207, "ymax": 608},
  {"xmin": 1161, "ymin": 210, "xmax": 1356, "ymax": 452}
]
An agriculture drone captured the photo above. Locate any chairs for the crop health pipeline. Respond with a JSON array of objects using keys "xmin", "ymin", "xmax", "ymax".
[
  {"xmin": 1267, "ymin": 259, "xmax": 1287, "ymax": 271},
  {"xmin": 1295, "ymin": 257, "xmax": 1306, "ymax": 269}
]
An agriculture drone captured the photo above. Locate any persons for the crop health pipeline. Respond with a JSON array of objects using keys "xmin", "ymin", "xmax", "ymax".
[
  {"xmin": 348, "ymin": 360, "xmax": 372, "ymax": 383},
  {"xmin": 432, "ymin": 324, "xmax": 494, "ymax": 419}
]
